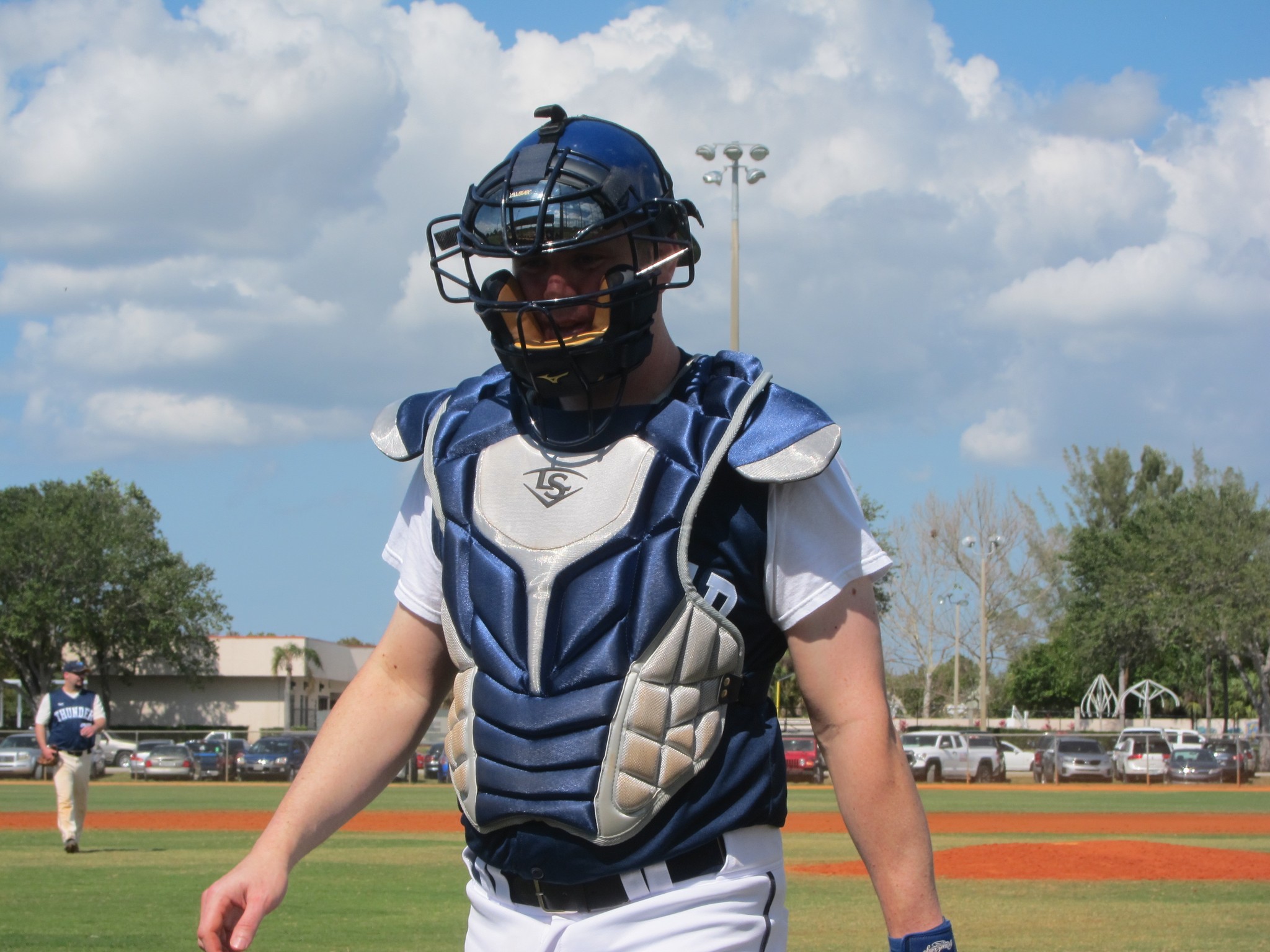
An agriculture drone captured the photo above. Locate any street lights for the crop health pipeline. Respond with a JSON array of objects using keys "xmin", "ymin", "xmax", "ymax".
[
  {"xmin": 961, "ymin": 535, "xmax": 1003, "ymax": 731},
  {"xmin": 696, "ymin": 139, "xmax": 770, "ymax": 348},
  {"xmin": 938, "ymin": 592, "xmax": 969, "ymax": 717}
]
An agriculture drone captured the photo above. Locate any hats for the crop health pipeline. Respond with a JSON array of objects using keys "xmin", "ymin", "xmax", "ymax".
[{"xmin": 63, "ymin": 662, "xmax": 88, "ymax": 674}]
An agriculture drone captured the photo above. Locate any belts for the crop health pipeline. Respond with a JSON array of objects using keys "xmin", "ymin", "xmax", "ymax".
[
  {"xmin": 473, "ymin": 836, "xmax": 727, "ymax": 912},
  {"xmin": 66, "ymin": 749, "xmax": 92, "ymax": 757}
]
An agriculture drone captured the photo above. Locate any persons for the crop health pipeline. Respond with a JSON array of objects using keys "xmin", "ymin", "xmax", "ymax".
[
  {"xmin": 35, "ymin": 660, "xmax": 108, "ymax": 854},
  {"xmin": 195, "ymin": 111, "xmax": 971, "ymax": 951}
]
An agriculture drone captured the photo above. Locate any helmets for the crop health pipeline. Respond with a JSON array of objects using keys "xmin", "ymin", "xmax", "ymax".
[{"xmin": 426, "ymin": 104, "xmax": 705, "ymax": 452}]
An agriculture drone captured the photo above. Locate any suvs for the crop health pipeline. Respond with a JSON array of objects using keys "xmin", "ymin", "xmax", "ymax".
[{"xmin": 781, "ymin": 729, "xmax": 830, "ymax": 784}]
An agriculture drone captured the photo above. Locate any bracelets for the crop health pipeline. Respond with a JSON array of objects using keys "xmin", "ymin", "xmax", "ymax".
[{"xmin": 887, "ymin": 917, "xmax": 956, "ymax": 952}]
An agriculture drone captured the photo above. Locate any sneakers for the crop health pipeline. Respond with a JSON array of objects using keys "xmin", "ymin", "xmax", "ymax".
[{"xmin": 65, "ymin": 840, "xmax": 79, "ymax": 852}]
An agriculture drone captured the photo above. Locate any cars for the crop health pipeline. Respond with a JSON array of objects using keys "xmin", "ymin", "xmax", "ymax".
[
  {"xmin": 437, "ymin": 748, "xmax": 452, "ymax": 783},
  {"xmin": 423, "ymin": 742, "xmax": 446, "ymax": 777},
  {"xmin": 0, "ymin": 732, "xmax": 50, "ymax": 781},
  {"xmin": 145, "ymin": 743, "xmax": 201, "ymax": 782},
  {"xmin": 129, "ymin": 738, "xmax": 177, "ymax": 778},
  {"xmin": 1040, "ymin": 737, "xmax": 1115, "ymax": 787},
  {"xmin": 900, "ymin": 729, "xmax": 1054, "ymax": 784},
  {"xmin": 1106, "ymin": 726, "xmax": 1259, "ymax": 784},
  {"xmin": 415, "ymin": 752, "xmax": 424, "ymax": 769},
  {"xmin": 48, "ymin": 728, "xmax": 252, "ymax": 781},
  {"xmin": 237, "ymin": 734, "xmax": 310, "ymax": 784}
]
